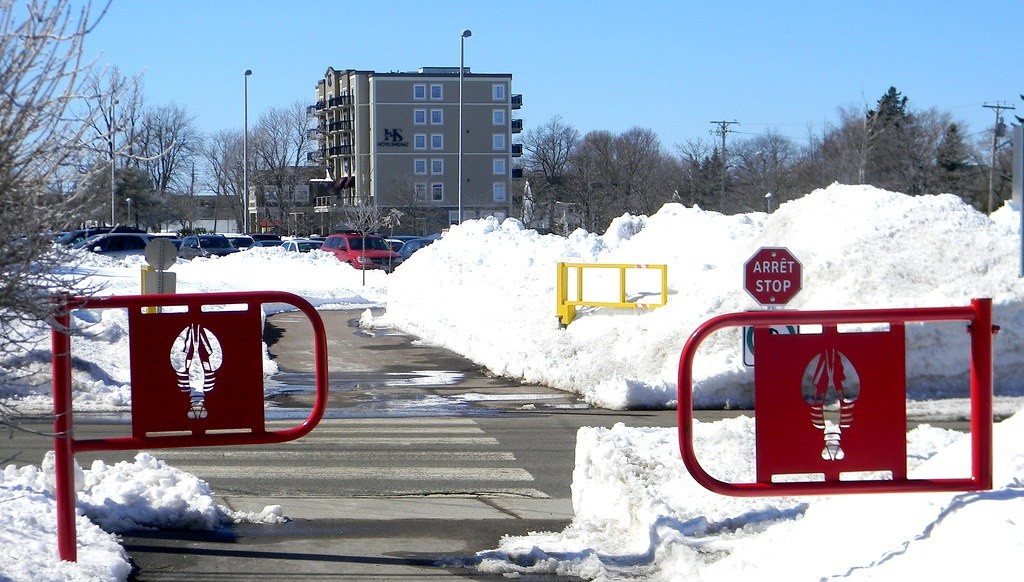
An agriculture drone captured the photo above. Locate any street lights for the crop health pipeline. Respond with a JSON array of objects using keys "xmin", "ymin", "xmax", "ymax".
[
  {"xmin": 458, "ymin": 30, "xmax": 472, "ymax": 226},
  {"xmin": 111, "ymin": 98, "xmax": 119, "ymax": 227},
  {"xmin": 243, "ymin": 69, "xmax": 253, "ymax": 233}
]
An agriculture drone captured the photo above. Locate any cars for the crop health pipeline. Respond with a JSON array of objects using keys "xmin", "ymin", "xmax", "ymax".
[
  {"xmin": 394, "ymin": 238, "xmax": 435, "ymax": 263},
  {"xmin": 39, "ymin": 223, "xmax": 420, "ymax": 262}
]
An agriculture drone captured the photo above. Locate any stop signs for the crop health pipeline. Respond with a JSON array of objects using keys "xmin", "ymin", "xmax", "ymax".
[{"xmin": 742, "ymin": 247, "xmax": 803, "ymax": 305}]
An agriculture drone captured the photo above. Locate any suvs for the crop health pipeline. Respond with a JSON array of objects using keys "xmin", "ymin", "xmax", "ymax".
[{"xmin": 321, "ymin": 233, "xmax": 403, "ymax": 273}]
